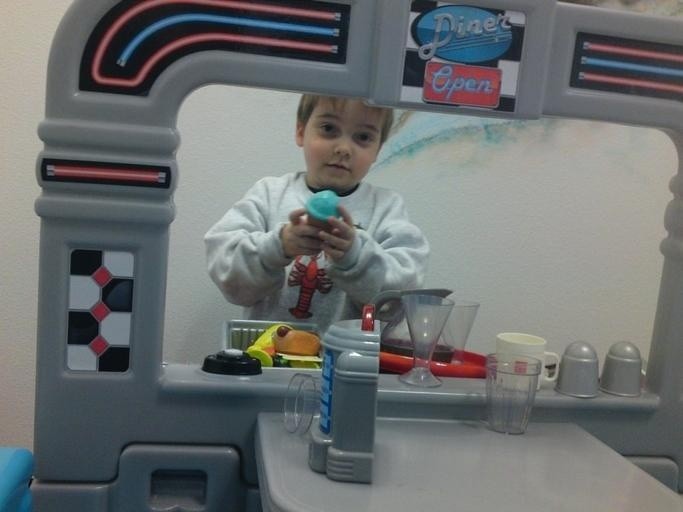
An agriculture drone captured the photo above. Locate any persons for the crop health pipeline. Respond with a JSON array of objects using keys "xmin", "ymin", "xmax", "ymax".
[{"xmin": 200, "ymin": 89, "xmax": 432, "ymax": 346}]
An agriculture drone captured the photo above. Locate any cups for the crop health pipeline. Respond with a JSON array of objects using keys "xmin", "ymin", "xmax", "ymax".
[
  {"xmin": 493, "ymin": 332, "xmax": 560, "ymax": 393},
  {"xmin": 279, "ymin": 372, "xmax": 323, "ymax": 438},
  {"xmin": 486, "ymin": 352, "xmax": 541, "ymax": 436},
  {"xmin": 441, "ymin": 299, "xmax": 482, "ymax": 353}
]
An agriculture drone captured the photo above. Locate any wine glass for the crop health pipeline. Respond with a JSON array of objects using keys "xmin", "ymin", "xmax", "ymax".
[{"xmin": 397, "ymin": 294, "xmax": 454, "ymax": 389}]
